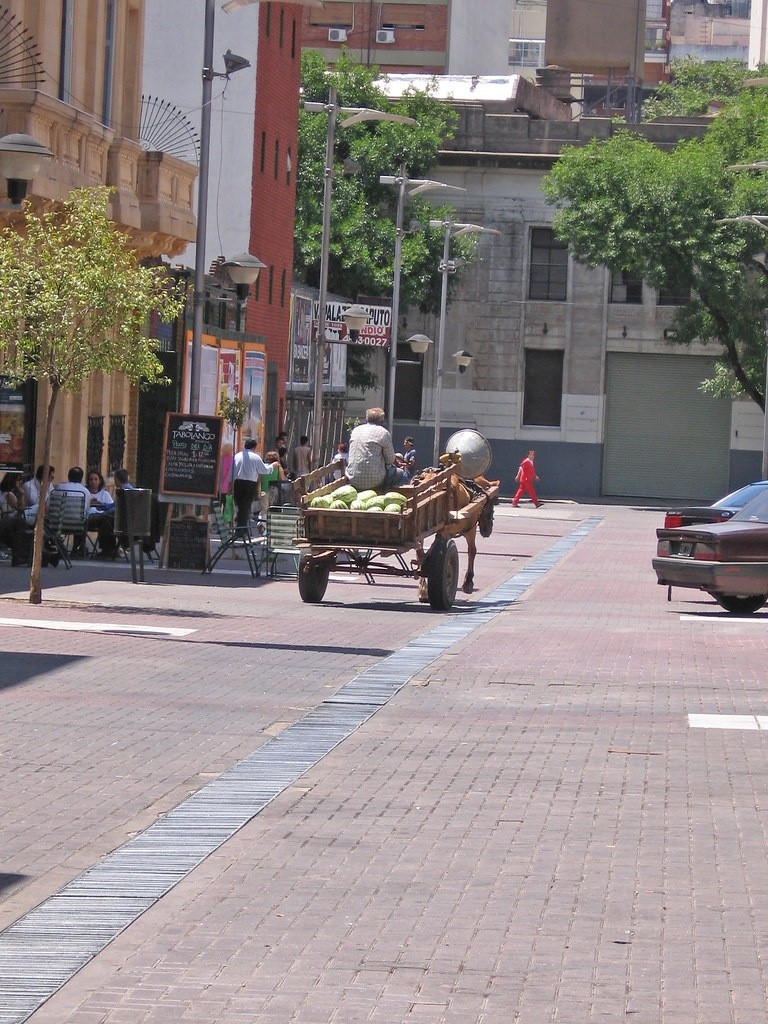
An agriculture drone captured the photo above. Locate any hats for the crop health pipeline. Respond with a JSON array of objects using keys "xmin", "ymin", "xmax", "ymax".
[
  {"xmin": 395, "ymin": 453, "xmax": 403, "ymax": 459},
  {"xmin": 405, "ymin": 437, "xmax": 414, "ymax": 445}
]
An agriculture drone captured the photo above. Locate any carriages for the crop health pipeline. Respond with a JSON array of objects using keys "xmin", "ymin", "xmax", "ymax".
[{"xmin": 287, "ymin": 452, "xmax": 501, "ymax": 610}]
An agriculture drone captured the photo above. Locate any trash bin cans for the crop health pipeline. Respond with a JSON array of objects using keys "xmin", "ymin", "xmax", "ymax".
[{"xmin": 114, "ymin": 488, "xmax": 151, "ymax": 537}]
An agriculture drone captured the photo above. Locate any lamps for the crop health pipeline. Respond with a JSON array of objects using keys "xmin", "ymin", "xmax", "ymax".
[{"xmin": 665, "ymin": 328, "xmax": 678, "ymax": 338}]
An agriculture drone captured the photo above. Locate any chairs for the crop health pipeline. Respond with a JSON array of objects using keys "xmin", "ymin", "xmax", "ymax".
[
  {"xmin": 202, "ymin": 500, "xmax": 308, "ymax": 579},
  {"xmin": 0, "ymin": 489, "xmax": 163, "ymax": 567}
]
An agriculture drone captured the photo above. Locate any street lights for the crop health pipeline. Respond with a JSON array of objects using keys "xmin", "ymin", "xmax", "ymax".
[
  {"xmin": 304, "ymin": 83, "xmax": 423, "ymax": 468},
  {"xmin": 376, "ymin": 159, "xmax": 469, "ymax": 451},
  {"xmin": 429, "ymin": 216, "xmax": 503, "ymax": 469}
]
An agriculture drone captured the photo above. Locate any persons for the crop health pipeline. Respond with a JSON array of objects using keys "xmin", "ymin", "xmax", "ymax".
[
  {"xmin": 219, "ymin": 432, "xmax": 313, "ymax": 541},
  {"xmin": 345, "ymin": 408, "xmax": 404, "ymax": 494},
  {"xmin": 395, "ymin": 436, "xmax": 416, "ymax": 480},
  {"xmin": 333, "ymin": 444, "xmax": 349, "ymax": 483},
  {"xmin": 511, "ymin": 451, "xmax": 544, "ymax": 508},
  {"xmin": 0, "ymin": 464, "xmax": 135, "ymax": 566}
]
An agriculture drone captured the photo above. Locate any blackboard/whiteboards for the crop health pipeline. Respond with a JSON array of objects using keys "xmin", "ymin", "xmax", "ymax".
[
  {"xmin": 158, "ymin": 411, "xmax": 224, "ymax": 497},
  {"xmin": 166, "ymin": 519, "xmax": 209, "ymax": 571}
]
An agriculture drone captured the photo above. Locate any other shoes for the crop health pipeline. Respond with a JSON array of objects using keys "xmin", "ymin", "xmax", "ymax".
[
  {"xmin": 536, "ymin": 504, "xmax": 544, "ymax": 508},
  {"xmin": 514, "ymin": 505, "xmax": 519, "ymax": 507}
]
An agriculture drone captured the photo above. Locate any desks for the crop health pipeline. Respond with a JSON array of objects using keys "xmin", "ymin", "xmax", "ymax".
[{"xmin": 83, "ymin": 501, "xmax": 114, "ymax": 559}]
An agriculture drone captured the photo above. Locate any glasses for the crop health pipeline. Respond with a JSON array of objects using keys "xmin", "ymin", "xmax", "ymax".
[{"xmin": 17, "ymin": 480, "xmax": 22, "ymax": 483}]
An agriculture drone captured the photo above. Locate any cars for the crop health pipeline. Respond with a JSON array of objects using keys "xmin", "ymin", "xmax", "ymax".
[
  {"xmin": 664, "ymin": 479, "xmax": 768, "ymax": 531},
  {"xmin": 650, "ymin": 487, "xmax": 768, "ymax": 614}
]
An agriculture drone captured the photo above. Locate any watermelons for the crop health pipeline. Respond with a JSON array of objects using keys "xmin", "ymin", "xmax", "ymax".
[{"xmin": 311, "ymin": 485, "xmax": 407, "ymax": 512}]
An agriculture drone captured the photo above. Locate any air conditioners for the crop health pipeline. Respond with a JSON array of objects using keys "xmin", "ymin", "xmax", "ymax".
[
  {"xmin": 376, "ymin": 30, "xmax": 394, "ymax": 44},
  {"xmin": 328, "ymin": 29, "xmax": 347, "ymax": 42}
]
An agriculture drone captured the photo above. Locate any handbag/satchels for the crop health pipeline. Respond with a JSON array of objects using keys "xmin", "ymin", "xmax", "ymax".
[{"xmin": 223, "ymin": 493, "xmax": 233, "ymax": 522}]
